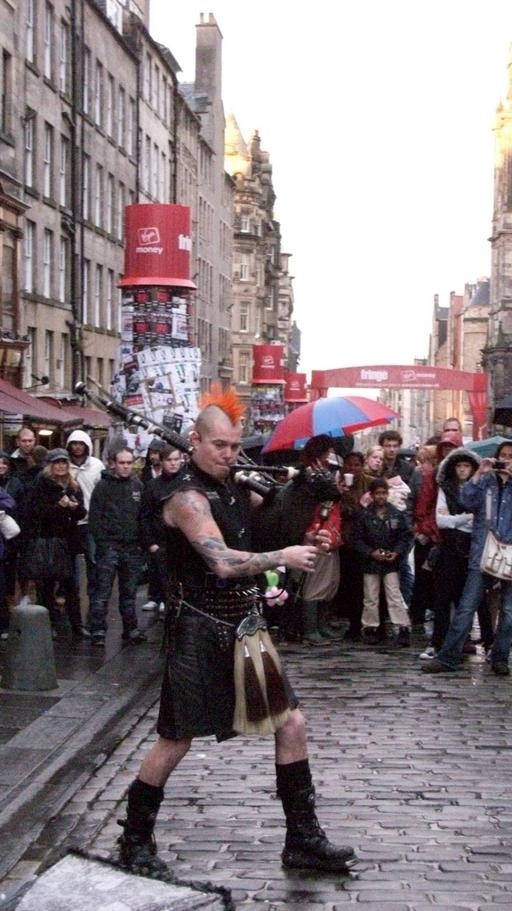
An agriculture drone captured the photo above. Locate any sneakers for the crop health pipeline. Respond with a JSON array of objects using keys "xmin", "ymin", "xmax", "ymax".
[
  {"xmin": 4, "ymin": 624, "xmax": 149, "ymax": 648},
  {"xmin": 141, "ymin": 598, "xmax": 165, "ymax": 614}
]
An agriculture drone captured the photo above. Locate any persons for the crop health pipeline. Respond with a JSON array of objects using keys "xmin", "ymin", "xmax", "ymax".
[
  {"xmin": 113, "ymin": 385, "xmax": 358, "ymax": 880},
  {"xmin": 0, "ymin": 419, "xmax": 510, "ymax": 675}
]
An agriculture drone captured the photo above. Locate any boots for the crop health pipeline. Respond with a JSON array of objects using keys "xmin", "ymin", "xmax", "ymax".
[
  {"xmin": 277, "ymin": 784, "xmax": 359, "ymax": 872},
  {"xmin": 117, "ymin": 794, "xmax": 171, "ymax": 877}
]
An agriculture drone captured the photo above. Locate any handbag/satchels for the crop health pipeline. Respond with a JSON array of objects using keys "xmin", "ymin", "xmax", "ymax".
[
  {"xmin": 433, "ymin": 538, "xmax": 470, "ymax": 588},
  {"xmin": 480, "ymin": 528, "xmax": 512, "ymax": 582},
  {"xmin": 2, "ymin": 514, "xmax": 21, "ymax": 540}
]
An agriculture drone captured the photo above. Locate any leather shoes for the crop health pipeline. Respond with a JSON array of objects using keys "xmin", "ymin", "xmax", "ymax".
[
  {"xmin": 303, "ymin": 621, "xmax": 427, "ymax": 647},
  {"xmin": 420, "ymin": 635, "xmax": 512, "ymax": 675}
]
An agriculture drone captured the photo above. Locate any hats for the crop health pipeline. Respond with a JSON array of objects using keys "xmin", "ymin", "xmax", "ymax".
[{"xmin": 45, "ymin": 448, "xmax": 69, "ymax": 462}]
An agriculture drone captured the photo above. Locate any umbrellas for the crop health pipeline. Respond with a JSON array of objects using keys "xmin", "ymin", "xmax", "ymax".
[{"xmin": 261, "ymin": 395, "xmax": 405, "ymax": 483}]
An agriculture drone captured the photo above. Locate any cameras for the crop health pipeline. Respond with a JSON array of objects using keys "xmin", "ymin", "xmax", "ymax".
[{"xmin": 492, "ymin": 461, "xmax": 506, "ymax": 469}]
